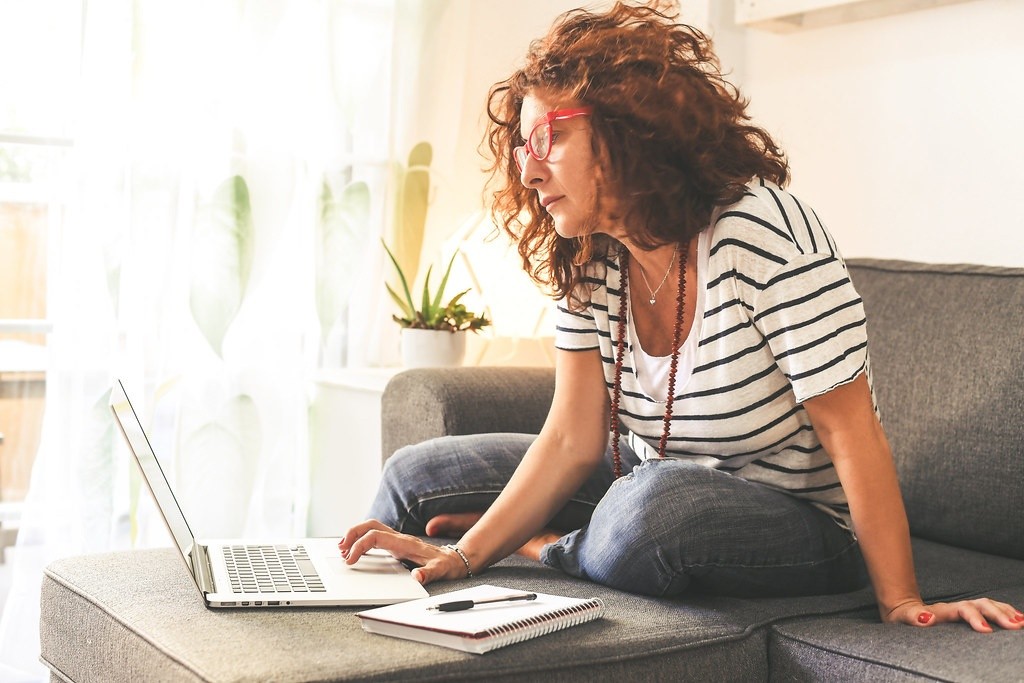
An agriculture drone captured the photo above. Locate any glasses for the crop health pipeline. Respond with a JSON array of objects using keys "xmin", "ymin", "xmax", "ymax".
[{"xmin": 513, "ymin": 104, "xmax": 594, "ymax": 173}]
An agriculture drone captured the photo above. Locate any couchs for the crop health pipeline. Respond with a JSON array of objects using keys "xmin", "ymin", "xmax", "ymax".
[{"xmin": 43, "ymin": 260, "xmax": 1024, "ymax": 683}]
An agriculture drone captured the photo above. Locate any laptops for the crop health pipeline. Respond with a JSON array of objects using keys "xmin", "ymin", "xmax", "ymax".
[{"xmin": 109, "ymin": 377, "xmax": 430, "ymax": 611}]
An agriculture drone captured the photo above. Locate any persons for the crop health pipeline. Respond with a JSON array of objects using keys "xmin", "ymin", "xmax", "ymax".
[{"xmin": 339, "ymin": 0, "xmax": 1024, "ymax": 632}]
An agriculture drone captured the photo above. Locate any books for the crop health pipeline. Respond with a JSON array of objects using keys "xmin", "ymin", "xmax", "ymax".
[{"xmin": 355, "ymin": 585, "xmax": 606, "ymax": 655}]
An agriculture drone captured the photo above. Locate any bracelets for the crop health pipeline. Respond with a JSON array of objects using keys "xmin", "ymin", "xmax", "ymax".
[{"xmin": 447, "ymin": 544, "xmax": 473, "ymax": 578}]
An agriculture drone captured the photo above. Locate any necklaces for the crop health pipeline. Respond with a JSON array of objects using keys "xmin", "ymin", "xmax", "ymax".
[
  {"xmin": 611, "ymin": 241, "xmax": 690, "ymax": 479},
  {"xmin": 639, "ymin": 242, "xmax": 680, "ymax": 306}
]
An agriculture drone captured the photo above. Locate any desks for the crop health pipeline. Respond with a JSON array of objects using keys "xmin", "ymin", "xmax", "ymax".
[{"xmin": 308, "ymin": 369, "xmax": 423, "ymax": 537}]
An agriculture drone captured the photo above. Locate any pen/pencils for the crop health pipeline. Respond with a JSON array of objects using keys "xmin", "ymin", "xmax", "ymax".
[{"xmin": 426, "ymin": 593, "xmax": 537, "ymax": 612}]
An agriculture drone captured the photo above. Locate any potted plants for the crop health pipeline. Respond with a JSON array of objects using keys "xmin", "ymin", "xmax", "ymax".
[{"xmin": 383, "ymin": 240, "xmax": 491, "ymax": 370}]
